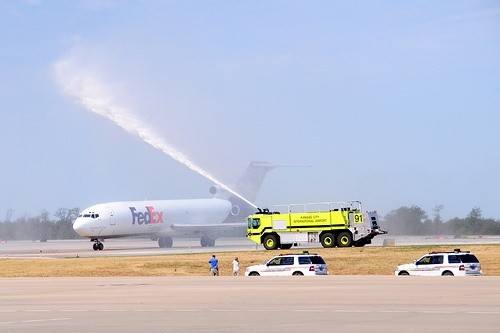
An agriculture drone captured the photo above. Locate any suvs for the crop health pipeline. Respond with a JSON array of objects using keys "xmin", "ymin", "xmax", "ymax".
[
  {"xmin": 394, "ymin": 249, "xmax": 483, "ymax": 276},
  {"xmin": 245, "ymin": 249, "xmax": 329, "ymax": 276}
]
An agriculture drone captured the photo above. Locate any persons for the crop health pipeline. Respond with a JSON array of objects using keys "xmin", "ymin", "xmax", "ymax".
[
  {"xmin": 232, "ymin": 257, "xmax": 240, "ymax": 276},
  {"xmin": 208, "ymin": 254, "xmax": 219, "ymax": 276}
]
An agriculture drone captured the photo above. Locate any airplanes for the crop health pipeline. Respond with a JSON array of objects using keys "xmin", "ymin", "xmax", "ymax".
[{"xmin": 72, "ymin": 160, "xmax": 274, "ymax": 250}]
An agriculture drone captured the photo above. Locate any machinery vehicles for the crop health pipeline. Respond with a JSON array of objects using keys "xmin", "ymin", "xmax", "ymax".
[{"xmin": 244, "ymin": 199, "xmax": 388, "ymax": 250}]
